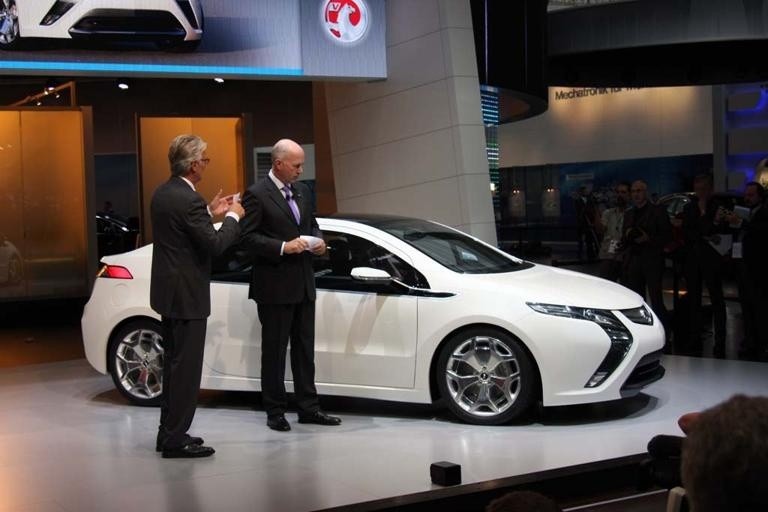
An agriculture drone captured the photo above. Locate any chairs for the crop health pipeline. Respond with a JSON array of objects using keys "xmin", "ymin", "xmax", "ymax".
[
  {"xmin": 350, "ymin": 242, "xmax": 371, "ymax": 267},
  {"xmin": 327, "ymin": 237, "xmax": 354, "ymax": 277}
]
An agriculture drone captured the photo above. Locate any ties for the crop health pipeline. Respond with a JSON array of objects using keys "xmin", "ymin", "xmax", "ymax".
[{"xmin": 282, "ymin": 186, "xmax": 300, "ymax": 225}]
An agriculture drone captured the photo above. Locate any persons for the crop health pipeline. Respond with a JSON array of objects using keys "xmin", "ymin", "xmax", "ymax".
[
  {"xmin": 150, "ymin": 134, "xmax": 245, "ymax": 458},
  {"xmin": 680, "ymin": 394, "xmax": 768, "ymax": 512},
  {"xmin": 104, "ymin": 201, "xmax": 114, "ymax": 213},
  {"xmin": 574, "ymin": 181, "xmax": 768, "ymax": 354},
  {"xmin": 485, "ymin": 490, "xmax": 562, "ymax": 512},
  {"xmin": 118, "ymin": 216, "xmax": 139, "ymax": 251},
  {"xmin": 241, "ymin": 138, "xmax": 342, "ymax": 431},
  {"xmin": 104, "ymin": 222, "xmax": 116, "ymax": 232}
]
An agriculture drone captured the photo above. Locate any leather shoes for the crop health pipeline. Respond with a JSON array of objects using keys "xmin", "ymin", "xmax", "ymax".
[
  {"xmin": 298, "ymin": 407, "xmax": 342, "ymax": 424},
  {"xmin": 266, "ymin": 414, "xmax": 290, "ymax": 431},
  {"xmin": 157, "ymin": 433, "xmax": 215, "ymax": 457}
]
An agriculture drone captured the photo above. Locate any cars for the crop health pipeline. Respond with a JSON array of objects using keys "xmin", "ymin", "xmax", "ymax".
[
  {"xmin": 90, "ymin": 215, "xmax": 140, "ymax": 255},
  {"xmin": 649, "ymin": 193, "xmax": 749, "ymax": 265},
  {"xmin": 0, "ymin": 231, "xmax": 24, "ymax": 288},
  {"xmin": 78, "ymin": 211, "xmax": 669, "ymax": 425},
  {"xmin": 0, "ymin": 1, "xmax": 204, "ymax": 51}
]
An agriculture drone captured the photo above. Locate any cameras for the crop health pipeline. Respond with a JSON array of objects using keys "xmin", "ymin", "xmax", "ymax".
[{"xmin": 629, "ymin": 229, "xmax": 643, "ymax": 241}]
